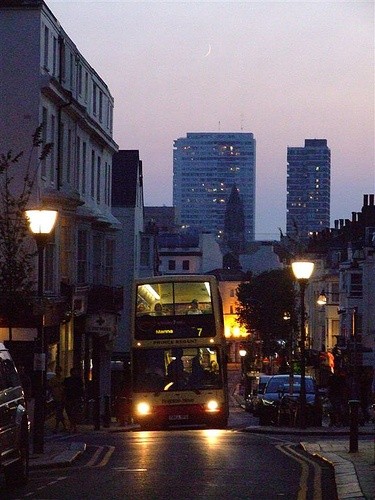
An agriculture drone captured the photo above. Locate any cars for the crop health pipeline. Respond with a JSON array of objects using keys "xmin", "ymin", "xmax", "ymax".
[
  {"xmin": 0, "ymin": 342, "xmax": 31, "ymax": 492},
  {"xmin": 245, "ymin": 371, "xmax": 262, "ymax": 415},
  {"xmin": 260, "ymin": 374, "xmax": 324, "ymax": 428},
  {"xmin": 258, "ymin": 376, "xmax": 272, "ymax": 406}
]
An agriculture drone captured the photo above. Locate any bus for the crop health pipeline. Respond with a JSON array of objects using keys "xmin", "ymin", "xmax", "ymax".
[{"xmin": 132, "ymin": 275, "xmax": 228, "ymax": 429}]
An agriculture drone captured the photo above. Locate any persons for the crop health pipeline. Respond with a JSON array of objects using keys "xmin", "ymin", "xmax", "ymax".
[
  {"xmin": 47, "ymin": 365, "xmax": 66, "ymax": 433},
  {"xmin": 187, "ymin": 300, "xmax": 203, "ymax": 315},
  {"xmin": 153, "ymin": 303, "xmax": 165, "ymax": 317},
  {"xmin": 63, "ymin": 368, "xmax": 81, "ymax": 428}
]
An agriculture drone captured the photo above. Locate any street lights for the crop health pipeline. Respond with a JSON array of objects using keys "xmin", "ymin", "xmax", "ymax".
[
  {"xmin": 290, "ymin": 261, "xmax": 315, "ymax": 426},
  {"xmin": 22, "ymin": 208, "xmax": 60, "ymax": 454},
  {"xmin": 238, "ymin": 350, "xmax": 246, "ymax": 371}
]
